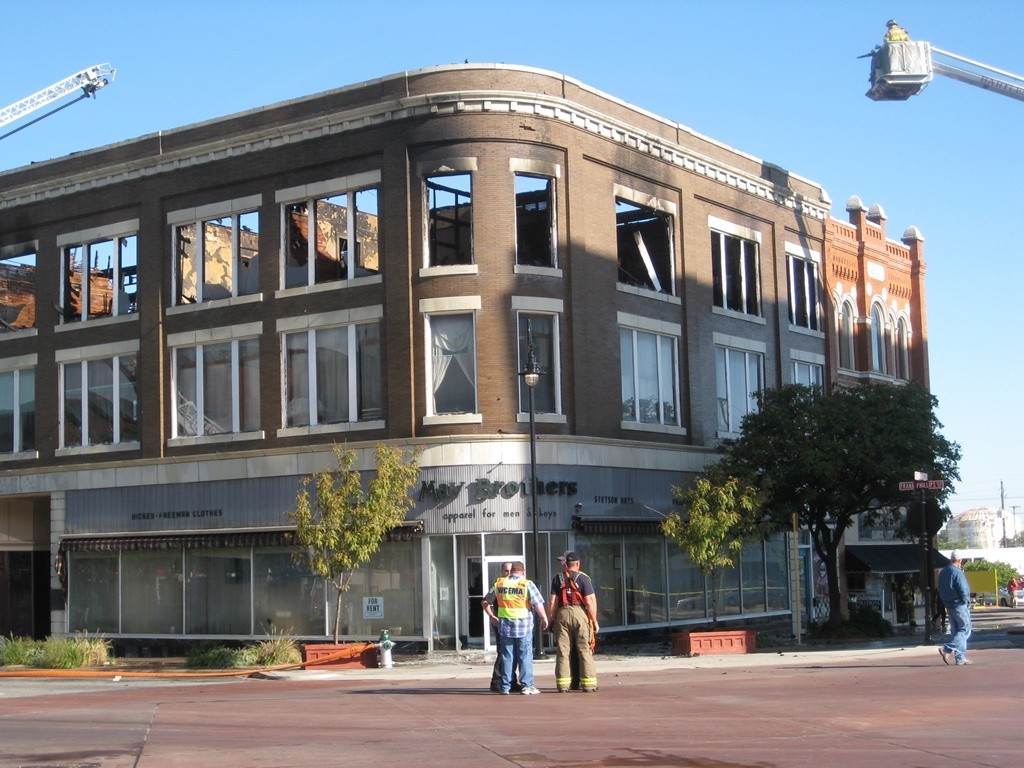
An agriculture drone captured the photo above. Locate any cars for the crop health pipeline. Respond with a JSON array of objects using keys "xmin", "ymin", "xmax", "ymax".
[{"xmin": 974, "ymin": 586, "xmax": 1024, "ymax": 606}]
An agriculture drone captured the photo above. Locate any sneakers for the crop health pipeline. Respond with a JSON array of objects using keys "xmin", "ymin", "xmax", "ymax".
[
  {"xmin": 521, "ymin": 687, "xmax": 541, "ymax": 694},
  {"xmin": 956, "ymin": 660, "xmax": 973, "ymax": 665},
  {"xmin": 940, "ymin": 648, "xmax": 950, "ymax": 665}
]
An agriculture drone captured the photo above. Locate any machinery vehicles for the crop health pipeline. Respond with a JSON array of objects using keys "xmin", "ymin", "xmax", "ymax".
[
  {"xmin": 865, "ymin": 41, "xmax": 1024, "ymax": 107},
  {"xmin": 0, "ymin": 61, "xmax": 116, "ymax": 144}
]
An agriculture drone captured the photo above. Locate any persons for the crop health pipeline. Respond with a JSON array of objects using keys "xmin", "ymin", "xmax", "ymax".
[
  {"xmin": 549, "ymin": 551, "xmax": 599, "ymax": 693},
  {"xmin": 898, "ymin": 574, "xmax": 919, "ymax": 628},
  {"xmin": 1007, "ymin": 576, "xmax": 1018, "ymax": 608},
  {"xmin": 882, "ymin": 19, "xmax": 910, "ymax": 44},
  {"xmin": 931, "ymin": 588, "xmax": 947, "ymax": 626},
  {"xmin": 481, "ymin": 561, "xmax": 549, "ymax": 696},
  {"xmin": 938, "ymin": 549, "xmax": 974, "ymax": 665}
]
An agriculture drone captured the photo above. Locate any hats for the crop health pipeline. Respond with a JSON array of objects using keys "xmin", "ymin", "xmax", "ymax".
[
  {"xmin": 951, "ymin": 549, "xmax": 962, "ymax": 560},
  {"xmin": 557, "ymin": 551, "xmax": 573, "ymax": 561},
  {"xmin": 567, "ymin": 552, "xmax": 582, "ymax": 561}
]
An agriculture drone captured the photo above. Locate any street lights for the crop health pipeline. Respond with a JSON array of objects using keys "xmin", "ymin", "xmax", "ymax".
[{"xmin": 518, "ymin": 318, "xmax": 549, "ymax": 661}]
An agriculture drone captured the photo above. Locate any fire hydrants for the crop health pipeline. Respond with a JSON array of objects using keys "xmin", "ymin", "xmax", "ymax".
[{"xmin": 375, "ymin": 629, "xmax": 396, "ymax": 668}]
